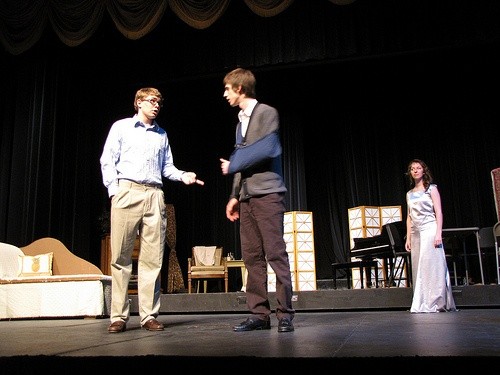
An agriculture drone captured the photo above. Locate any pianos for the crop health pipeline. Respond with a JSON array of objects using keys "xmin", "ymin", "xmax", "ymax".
[{"xmin": 349, "ymin": 220, "xmax": 456, "ymax": 289}]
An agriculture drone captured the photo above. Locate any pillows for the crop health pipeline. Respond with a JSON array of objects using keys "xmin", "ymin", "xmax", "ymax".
[{"xmin": 17, "ymin": 252, "xmax": 55, "ymax": 276}]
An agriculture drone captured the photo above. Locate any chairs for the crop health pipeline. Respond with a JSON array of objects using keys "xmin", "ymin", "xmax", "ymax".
[
  {"xmin": 442, "ymin": 249, "xmax": 468, "ymax": 284},
  {"xmin": 187, "ymin": 246, "xmax": 228, "ymax": 293},
  {"xmin": 385, "ymin": 224, "xmax": 410, "ymax": 286}
]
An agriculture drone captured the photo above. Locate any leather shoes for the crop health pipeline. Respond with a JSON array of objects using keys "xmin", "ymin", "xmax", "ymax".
[
  {"xmin": 108, "ymin": 321, "xmax": 127, "ymax": 333},
  {"xmin": 233, "ymin": 317, "xmax": 271, "ymax": 331},
  {"xmin": 141, "ymin": 320, "xmax": 164, "ymax": 331},
  {"xmin": 278, "ymin": 318, "xmax": 294, "ymax": 331}
]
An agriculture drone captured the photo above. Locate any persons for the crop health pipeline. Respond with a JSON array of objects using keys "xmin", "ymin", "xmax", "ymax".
[
  {"xmin": 100, "ymin": 87, "xmax": 204, "ymax": 333},
  {"xmin": 220, "ymin": 68, "xmax": 295, "ymax": 333},
  {"xmin": 405, "ymin": 158, "xmax": 459, "ymax": 313}
]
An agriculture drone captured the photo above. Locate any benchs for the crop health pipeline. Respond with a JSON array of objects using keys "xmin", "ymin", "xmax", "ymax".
[
  {"xmin": 332, "ymin": 260, "xmax": 378, "ymax": 289},
  {"xmin": 0, "ymin": 236, "xmax": 110, "ymax": 319}
]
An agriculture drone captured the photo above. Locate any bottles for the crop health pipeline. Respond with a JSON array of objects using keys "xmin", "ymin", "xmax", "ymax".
[{"xmin": 227, "ymin": 251, "xmax": 233, "ymax": 261}]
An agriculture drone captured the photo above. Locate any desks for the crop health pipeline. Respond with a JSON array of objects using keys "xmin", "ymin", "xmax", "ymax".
[
  {"xmin": 441, "ymin": 227, "xmax": 483, "ymax": 285},
  {"xmin": 224, "ymin": 260, "xmax": 246, "ymax": 292}
]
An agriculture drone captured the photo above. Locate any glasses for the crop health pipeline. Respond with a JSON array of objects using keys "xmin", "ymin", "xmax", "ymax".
[{"xmin": 141, "ymin": 100, "xmax": 163, "ymax": 108}]
